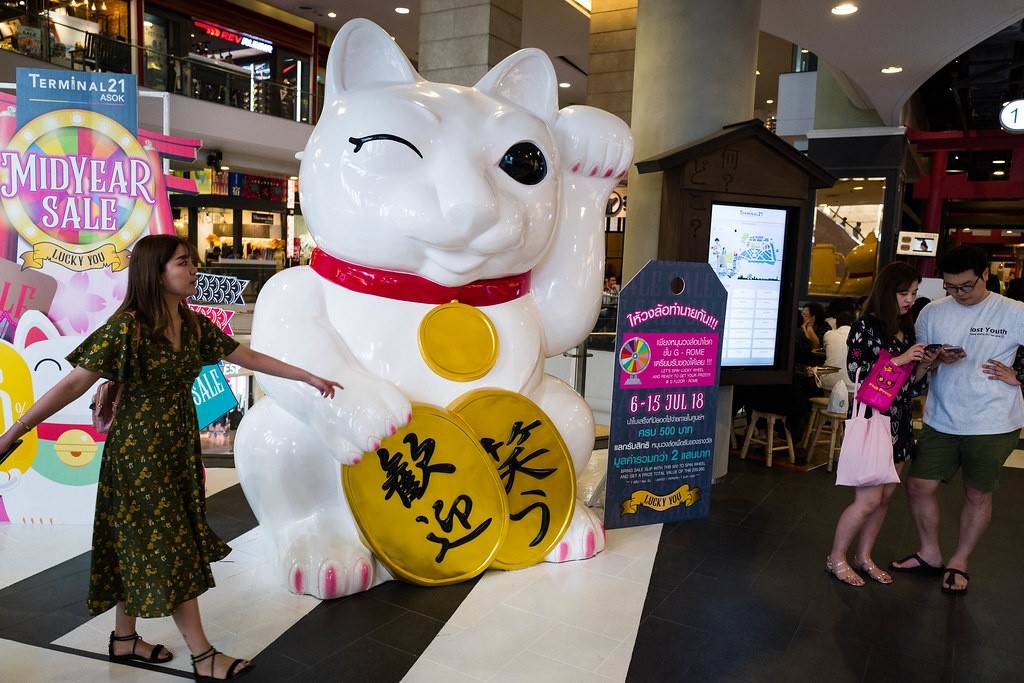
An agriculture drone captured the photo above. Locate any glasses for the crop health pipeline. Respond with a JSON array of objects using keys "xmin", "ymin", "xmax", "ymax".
[{"xmin": 943, "ymin": 276, "xmax": 980, "ymax": 293}]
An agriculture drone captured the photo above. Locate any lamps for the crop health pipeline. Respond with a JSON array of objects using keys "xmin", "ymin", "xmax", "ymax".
[{"xmin": 206, "ymin": 150, "xmax": 224, "ymax": 176}]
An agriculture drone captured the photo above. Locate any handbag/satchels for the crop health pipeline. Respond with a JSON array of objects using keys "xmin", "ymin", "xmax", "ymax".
[
  {"xmin": 835, "ymin": 368, "xmax": 901, "ymax": 486},
  {"xmin": 856, "ymin": 350, "xmax": 914, "ymax": 414}
]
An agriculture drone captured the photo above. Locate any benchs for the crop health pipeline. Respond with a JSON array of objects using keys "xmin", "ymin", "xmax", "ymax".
[{"xmin": 69, "ymin": 32, "xmax": 131, "ymax": 75}]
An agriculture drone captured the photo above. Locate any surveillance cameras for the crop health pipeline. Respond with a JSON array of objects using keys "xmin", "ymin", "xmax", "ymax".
[{"xmin": 212, "ymin": 161, "xmax": 224, "ymax": 176}]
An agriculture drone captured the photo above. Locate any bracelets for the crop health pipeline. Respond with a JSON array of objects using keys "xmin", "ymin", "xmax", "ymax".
[{"xmin": 16, "ymin": 420, "xmax": 31, "ymax": 431}]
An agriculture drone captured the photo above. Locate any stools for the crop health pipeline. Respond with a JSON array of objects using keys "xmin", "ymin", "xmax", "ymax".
[
  {"xmin": 802, "ymin": 397, "xmax": 832, "ymax": 448},
  {"xmin": 807, "ymin": 410, "xmax": 849, "ymax": 471},
  {"xmin": 741, "ymin": 411, "xmax": 795, "ymax": 467}
]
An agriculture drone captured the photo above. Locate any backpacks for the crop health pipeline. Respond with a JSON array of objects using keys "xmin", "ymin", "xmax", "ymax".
[{"xmin": 90, "ymin": 310, "xmax": 203, "ymax": 433}]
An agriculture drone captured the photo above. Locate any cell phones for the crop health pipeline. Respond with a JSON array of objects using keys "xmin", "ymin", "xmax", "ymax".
[
  {"xmin": 944, "ymin": 345, "xmax": 967, "ymax": 358},
  {"xmin": 917, "ymin": 343, "xmax": 943, "ymax": 358}
]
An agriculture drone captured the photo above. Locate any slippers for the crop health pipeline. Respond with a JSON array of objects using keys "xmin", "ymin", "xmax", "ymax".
[
  {"xmin": 944, "ymin": 567, "xmax": 969, "ymax": 594},
  {"xmin": 889, "ymin": 554, "xmax": 944, "ymax": 574}
]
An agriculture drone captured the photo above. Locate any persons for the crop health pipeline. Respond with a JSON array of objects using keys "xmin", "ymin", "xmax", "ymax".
[
  {"xmin": 891, "ymin": 243, "xmax": 1024, "ymax": 593},
  {"xmin": 0, "ymin": 233, "xmax": 345, "ymax": 682},
  {"xmin": 841, "ymin": 217, "xmax": 847, "ymax": 227},
  {"xmin": 272, "ymin": 247, "xmax": 286, "ymax": 274},
  {"xmin": 204, "ymin": 241, "xmax": 221, "ymax": 273},
  {"xmin": 853, "ymin": 222, "xmax": 862, "ymax": 240},
  {"xmin": 1005, "ymin": 277, "xmax": 1024, "ymax": 397},
  {"xmin": 824, "ymin": 260, "xmax": 938, "ymax": 588},
  {"xmin": 601, "ymin": 277, "xmax": 620, "ymax": 303},
  {"xmin": 747, "ymin": 300, "xmax": 862, "ymax": 450}
]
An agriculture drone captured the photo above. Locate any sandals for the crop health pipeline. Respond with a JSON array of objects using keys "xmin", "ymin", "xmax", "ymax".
[
  {"xmin": 191, "ymin": 645, "xmax": 256, "ymax": 683},
  {"xmin": 824, "ymin": 556, "xmax": 866, "ymax": 586},
  {"xmin": 850, "ymin": 555, "xmax": 893, "ymax": 583},
  {"xmin": 109, "ymin": 631, "xmax": 173, "ymax": 663}
]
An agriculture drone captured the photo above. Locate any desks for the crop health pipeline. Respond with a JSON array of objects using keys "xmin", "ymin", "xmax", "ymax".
[{"xmin": 174, "ymin": 53, "xmax": 256, "ymax": 113}]
